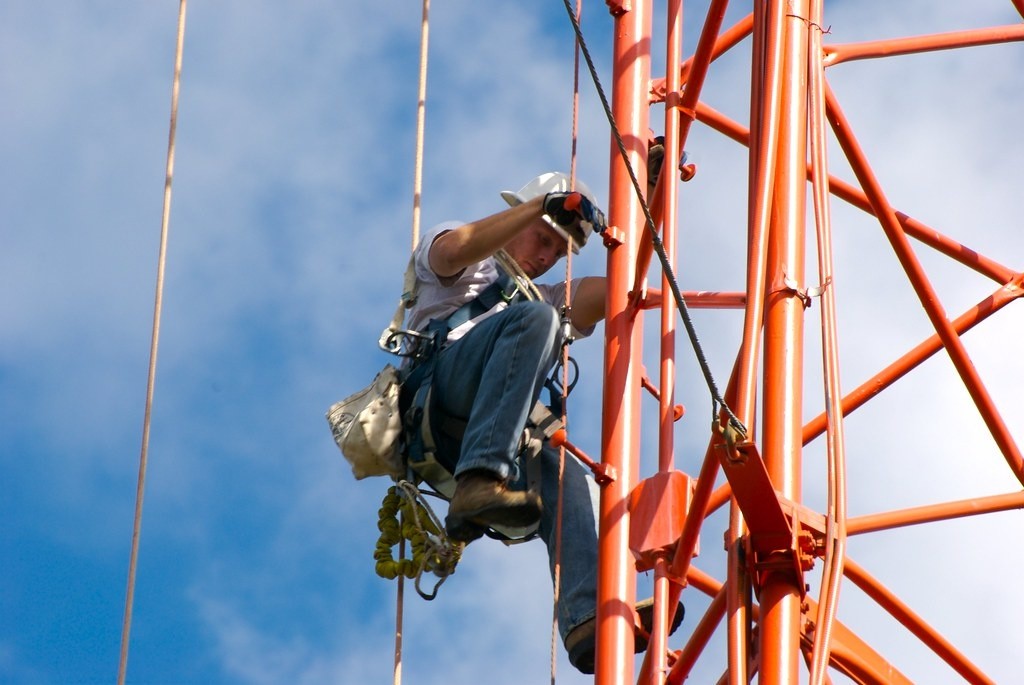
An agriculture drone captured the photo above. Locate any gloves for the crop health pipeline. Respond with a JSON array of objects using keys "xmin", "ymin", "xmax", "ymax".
[
  {"xmin": 542, "ymin": 191, "xmax": 604, "ymax": 233},
  {"xmin": 647, "ymin": 136, "xmax": 688, "ymax": 188}
]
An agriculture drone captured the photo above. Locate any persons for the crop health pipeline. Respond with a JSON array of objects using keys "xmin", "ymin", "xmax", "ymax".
[{"xmin": 399, "ymin": 171, "xmax": 685, "ymax": 674}]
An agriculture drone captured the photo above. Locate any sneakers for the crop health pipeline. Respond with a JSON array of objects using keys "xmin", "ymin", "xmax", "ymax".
[
  {"xmin": 445, "ymin": 470, "xmax": 543, "ymax": 545},
  {"xmin": 565, "ymin": 596, "xmax": 685, "ymax": 675}
]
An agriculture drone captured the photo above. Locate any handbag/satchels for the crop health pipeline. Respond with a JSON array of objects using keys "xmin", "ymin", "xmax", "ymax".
[{"xmin": 326, "ymin": 363, "xmax": 406, "ymax": 481}]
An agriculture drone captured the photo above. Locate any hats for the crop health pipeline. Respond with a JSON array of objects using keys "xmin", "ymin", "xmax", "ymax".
[{"xmin": 500, "ymin": 172, "xmax": 598, "ymax": 255}]
